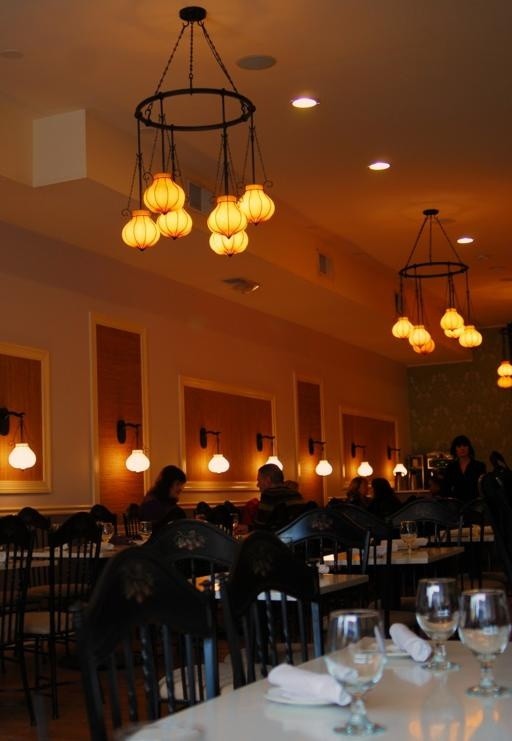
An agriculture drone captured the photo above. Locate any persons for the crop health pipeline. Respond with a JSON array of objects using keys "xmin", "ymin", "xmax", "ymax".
[
  {"xmin": 234, "ymin": 465, "xmax": 321, "ymax": 561},
  {"xmin": 345, "ymin": 477, "xmax": 403, "ymax": 540},
  {"xmin": 428, "ymin": 436, "xmax": 512, "ymax": 532},
  {"xmin": 136, "ymin": 465, "xmax": 187, "ymax": 532}
]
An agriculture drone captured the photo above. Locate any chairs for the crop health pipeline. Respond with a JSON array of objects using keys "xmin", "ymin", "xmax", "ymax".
[{"xmin": 0, "ymin": 484, "xmax": 511, "ymax": 741}]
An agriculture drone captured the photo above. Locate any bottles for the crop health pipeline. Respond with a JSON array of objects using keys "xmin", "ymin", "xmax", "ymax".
[{"xmin": 394, "ymin": 472, "xmax": 404, "ymax": 492}]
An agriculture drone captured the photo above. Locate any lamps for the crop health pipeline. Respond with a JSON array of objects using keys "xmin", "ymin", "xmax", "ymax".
[
  {"xmin": 385, "ymin": 206, "xmax": 483, "ymax": 355},
  {"xmin": 198, "ymin": 427, "xmax": 232, "ymax": 474},
  {"xmin": 1, "ymin": 401, "xmax": 42, "ymax": 474},
  {"xmin": 494, "ymin": 319, "xmax": 512, "ymax": 395},
  {"xmin": 115, "ymin": 417, "xmax": 150, "ymax": 474},
  {"xmin": 307, "ymin": 436, "xmax": 333, "ymax": 477},
  {"xmin": 255, "ymin": 431, "xmax": 284, "ymax": 473},
  {"xmin": 386, "ymin": 445, "xmax": 408, "ymax": 477},
  {"xmin": 350, "ymin": 441, "xmax": 375, "ymax": 479},
  {"xmin": 111, "ymin": 3, "xmax": 279, "ymax": 260}
]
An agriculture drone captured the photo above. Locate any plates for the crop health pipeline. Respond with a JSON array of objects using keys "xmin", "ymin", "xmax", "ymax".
[
  {"xmin": 259, "ymin": 686, "xmax": 336, "ymax": 709},
  {"xmin": 383, "ymin": 645, "xmax": 411, "ymax": 658}
]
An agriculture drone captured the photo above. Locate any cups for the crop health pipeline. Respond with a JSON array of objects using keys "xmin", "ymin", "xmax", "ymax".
[
  {"xmin": 101, "ymin": 522, "xmax": 115, "ymax": 545},
  {"xmin": 138, "ymin": 521, "xmax": 152, "ymax": 542}
]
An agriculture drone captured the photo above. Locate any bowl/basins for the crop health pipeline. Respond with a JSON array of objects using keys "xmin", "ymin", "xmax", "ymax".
[
  {"xmin": 380, "ymin": 541, "xmax": 398, "ymax": 553},
  {"xmin": 368, "ymin": 547, "xmax": 386, "ymax": 558}
]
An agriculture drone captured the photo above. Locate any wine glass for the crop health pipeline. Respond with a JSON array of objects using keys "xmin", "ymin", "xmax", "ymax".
[
  {"xmin": 413, "ymin": 578, "xmax": 465, "ymax": 672},
  {"xmin": 321, "ymin": 611, "xmax": 389, "ymax": 737},
  {"xmin": 452, "ymin": 589, "xmax": 511, "ymax": 699},
  {"xmin": 398, "ymin": 520, "xmax": 419, "ymax": 556}
]
